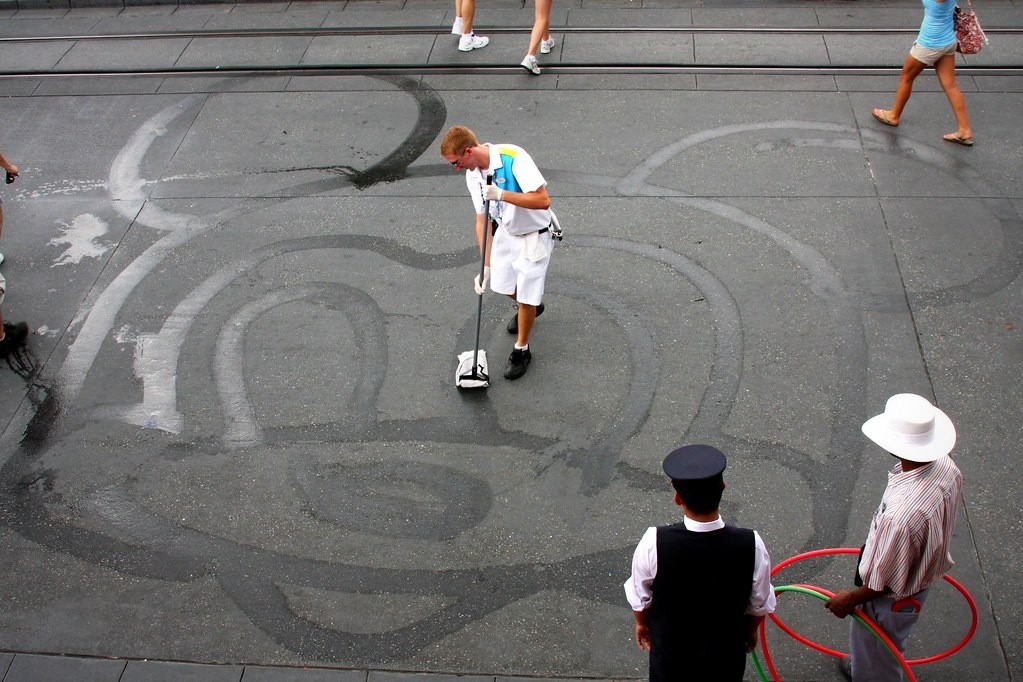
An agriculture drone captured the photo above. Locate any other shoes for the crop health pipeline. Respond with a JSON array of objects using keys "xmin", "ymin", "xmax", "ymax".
[
  {"xmin": 0, "ymin": 322, "xmax": 30, "ymax": 358},
  {"xmin": 840, "ymin": 656, "xmax": 852, "ymax": 682}
]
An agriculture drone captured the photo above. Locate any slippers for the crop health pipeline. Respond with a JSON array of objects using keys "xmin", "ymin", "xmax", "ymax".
[
  {"xmin": 943, "ymin": 133, "xmax": 973, "ymax": 146},
  {"xmin": 873, "ymin": 108, "xmax": 899, "ymax": 126}
]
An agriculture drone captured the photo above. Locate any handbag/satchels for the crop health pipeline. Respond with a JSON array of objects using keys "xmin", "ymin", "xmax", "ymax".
[{"xmin": 953, "ymin": 0, "xmax": 989, "ymax": 54}]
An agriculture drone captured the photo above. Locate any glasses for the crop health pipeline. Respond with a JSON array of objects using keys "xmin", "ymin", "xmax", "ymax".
[{"xmin": 451, "ymin": 146, "xmax": 471, "ymax": 167}]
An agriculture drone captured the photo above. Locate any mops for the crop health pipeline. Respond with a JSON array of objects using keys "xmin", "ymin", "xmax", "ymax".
[{"xmin": 454, "ymin": 173, "xmax": 493, "ymax": 389}]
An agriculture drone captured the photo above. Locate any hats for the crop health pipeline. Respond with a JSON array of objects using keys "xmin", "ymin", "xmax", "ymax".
[
  {"xmin": 662, "ymin": 445, "xmax": 726, "ymax": 488},
  {"xmin": 860, "ymin": 392, "xmax": 957, "ymax": 462}
]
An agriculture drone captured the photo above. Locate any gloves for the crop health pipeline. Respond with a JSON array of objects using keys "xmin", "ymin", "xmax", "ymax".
[
  {"xmin": 474, "ymin": 266, "xmax": 490, "ymax": 295},
  {"xmin": 482, "ymin": 182, "xmax": 503, "ymax": 201}
]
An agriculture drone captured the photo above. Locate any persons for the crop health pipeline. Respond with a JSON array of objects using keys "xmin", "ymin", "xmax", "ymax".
[
  {"xmin": 520, "ymin": 0, "xmax": 556, "ymax": 75},
  {"xmin": 439, "ymin": 126, "xmax": 555, "ymax": 383},
  {"xmin": 451, "ymin": 0, "xmax": 491, "ymax": 51},
  {"xmin": 0, "ymin": 152, "xmax": 29, "ymax": 357},
  {"xmin": 622, "ymin": 444, "xmax": 776, "ymax": 682},
  {"xmin": 824, "ymin": 392, "xmax": 962, "ymax": 682},
  {"xmin": 872, "ymin": 0, "xmax": 973, "ymax": 145}
]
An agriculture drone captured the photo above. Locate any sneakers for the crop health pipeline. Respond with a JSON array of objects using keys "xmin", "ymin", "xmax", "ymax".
[
  {"xmin": 451, "ymin": 23, "xmax": 474, "ymax": 36},
  {"xmin": 504, "ymin": 344, "xmax": 531, "ymax": 379},
  {"xmin": 540, "ymin": 39, "xmax": 554, "ymax": 53},
  {"xmin": 458, "ymin": 36, "xmax": 489, "ymax": 51},
  {"xmin": 521, "ymin": 56, "xmax": 541, "ymax": 74},
  {"xmin": 507, "ymin": 301, "xmax": 544, "ymax": 334}
]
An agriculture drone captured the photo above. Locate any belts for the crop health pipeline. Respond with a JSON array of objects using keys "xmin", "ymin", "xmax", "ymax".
[{"xmin": 538, "ymin": 222, "xmax": 552, "ymax": 234}]
